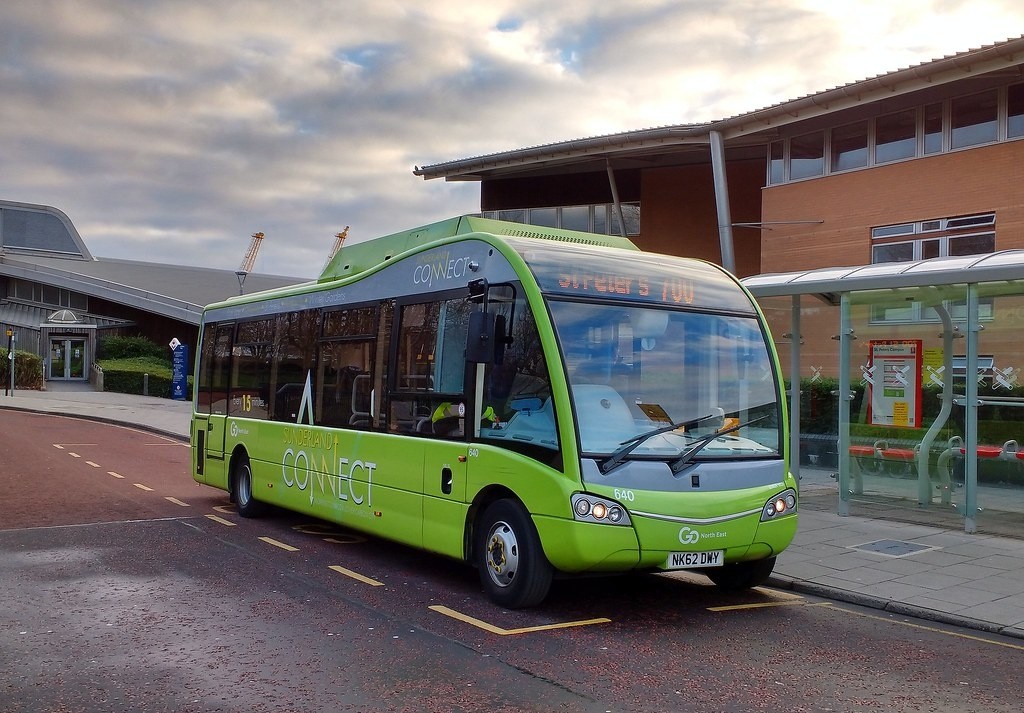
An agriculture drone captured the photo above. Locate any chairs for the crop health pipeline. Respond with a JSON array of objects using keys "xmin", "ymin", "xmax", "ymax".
[{"xmin": 225, "ymin": 361, "xmax": 372, "ymax": 423}]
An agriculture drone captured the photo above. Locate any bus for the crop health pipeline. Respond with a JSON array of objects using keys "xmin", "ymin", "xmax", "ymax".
[{"xmin": 188, "ymin": 212, "xmax": 798, "ymax": 613}]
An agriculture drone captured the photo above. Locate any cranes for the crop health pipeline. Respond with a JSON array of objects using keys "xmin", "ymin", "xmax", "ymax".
[
  {"xmin": 239, "ymin": 230, "xmax": 265, "ymax": 273},
  {"xmin": 317, "ymin": 225, "xmax": 350, "ymax": 279}
]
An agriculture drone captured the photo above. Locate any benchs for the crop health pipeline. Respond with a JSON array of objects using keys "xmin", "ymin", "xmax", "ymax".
[
  {"xmin": 849, "ymin": 441, "xmax": 934, "ymax": 506},
  {"xmin": 938, "ymin": 436, "xmax": 1023, "ymax": 510}
]
